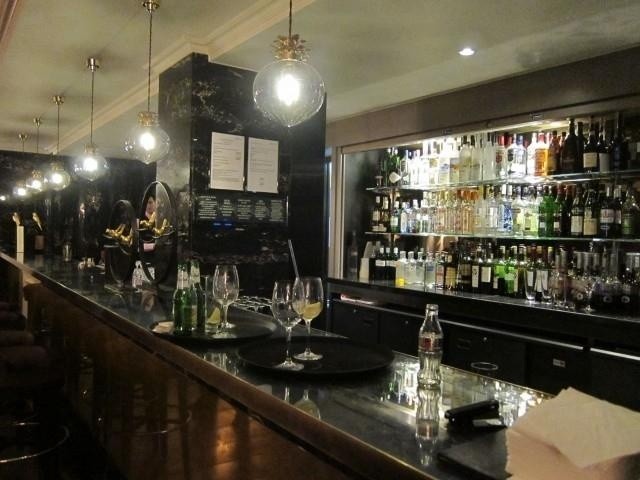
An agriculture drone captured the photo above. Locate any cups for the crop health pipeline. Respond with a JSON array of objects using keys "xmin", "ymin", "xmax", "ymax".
[
  {"xmin": 468, "ymin": 361, "xmax": 499, "ymax": 397},
  {"xmin": 62, "ymin": 245, "xmax": 73, "ymax": 262},
  {"xmin": 200, "ymin": 273, "xmax": 223, "ymax": 328}
]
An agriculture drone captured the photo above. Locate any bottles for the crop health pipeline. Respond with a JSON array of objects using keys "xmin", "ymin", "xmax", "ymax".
[
  {"xmin": 188, "ymin": 259, "xmax": 205, "ymax": 322},
  {"xmin": 376, "ymin": 111, "xmax": 639, "ymax": 187},
  {"xmin": 417, "ymin": 301, "xmax": 445, "ymax": 389},
  {"xmin": 374, "ymin": 241, "xmax": 640, "ymax": 317},
  {"xmin": 414, "ymin": 389, "xmax": 442, "ymax": 465},
  {"xmin": 346, "ymin": 229, "xmax": 358, "ymax": 274},
  {"xmin": 173, "ymin": 263, "xmax": 192, "ymax": 334},
  {"xmin": 367, "ymin": 186, "xmax": 640, "ymax": 237}
]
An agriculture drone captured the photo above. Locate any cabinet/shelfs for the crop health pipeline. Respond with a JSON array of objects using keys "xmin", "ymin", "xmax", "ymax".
[{"xmin": 326, "ymin": 47, "xmax": 640, "ymax": 411}]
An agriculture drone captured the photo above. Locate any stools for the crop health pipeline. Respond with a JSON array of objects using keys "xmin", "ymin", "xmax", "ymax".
[{"xmin": 0, "ymin": 301, "xmax": 48, "ymax": 463}]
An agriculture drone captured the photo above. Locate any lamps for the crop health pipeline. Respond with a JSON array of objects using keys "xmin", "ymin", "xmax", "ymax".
[
  {"xmin": 123, "ymin": 0, "xmax": 171, "ymax": 164},
  {"xmin": 252, "ymin": 1, "xmax": 325, "ymax": 129},
  {"xmin": 1, "ymin": 55, "xmax": 108, "ymax": 202}
]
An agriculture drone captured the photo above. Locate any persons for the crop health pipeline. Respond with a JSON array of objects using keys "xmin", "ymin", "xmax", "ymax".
[{"xmin": 141, "ymin": 195, "xmax": 160, "ymax": 251}]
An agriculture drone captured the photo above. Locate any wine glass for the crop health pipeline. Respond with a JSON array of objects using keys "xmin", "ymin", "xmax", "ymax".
[
  {"xmin": 291, "ymin": 274, "xmax": 326, "ymax": 360},
  {"xmin": 270, "ymin": 280, "xmax": 305, "ymax": 372},
  {"xmin": 213, "ymin": 263, "xmax": 239, "ymax": 329}
]
